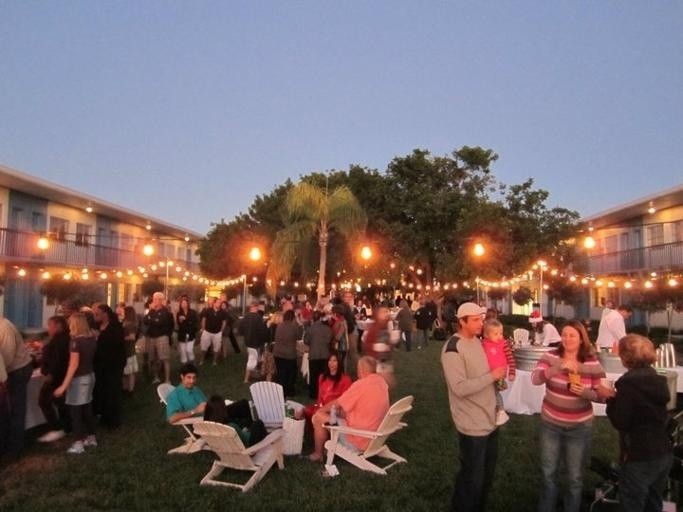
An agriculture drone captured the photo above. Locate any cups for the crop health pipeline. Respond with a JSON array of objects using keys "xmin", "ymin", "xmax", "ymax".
[{"xmin": 568, "ymin": 368, "xmax": 580, "ymax": 391}]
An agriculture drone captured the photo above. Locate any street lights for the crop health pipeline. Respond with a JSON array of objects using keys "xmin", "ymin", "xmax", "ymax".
[
  {"xmin": 536, "ymin": 256, "xmax": 548, "ymax": 317},
  {"xmin": 474, "ymin": 275, "xmax": 480, "ymax": 309}
]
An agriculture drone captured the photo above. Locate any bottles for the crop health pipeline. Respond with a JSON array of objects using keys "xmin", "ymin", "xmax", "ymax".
[
  {"xmin": 329, "ymin": 404, "xmax": 339, "ymax": 424},
  {"xmin": 611, "ymin": 341, "xmax": 618, "ymax": 354},
  {"xmin": 652, "ymin": 343, "xmax": 674, "ymax": 369}
]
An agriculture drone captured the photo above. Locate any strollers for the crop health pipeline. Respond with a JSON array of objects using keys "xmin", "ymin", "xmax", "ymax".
[{"xmin": 580, "ymin": 410, "xmax": 681, "ymax": 512}]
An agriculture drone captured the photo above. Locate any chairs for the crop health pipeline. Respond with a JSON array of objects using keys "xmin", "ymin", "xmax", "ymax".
[
  {"xmin": 157, "ymin": 382, "xmax": 234, "ymax": 455},
  {"xmin": 248, "ymin": 380, "xmax": 305, "ymax": 429},
  {"xmin": 513, "ymin": 328, "xmax": 530, "ymax": 348},
  {"xmin": 650, "ymin": 343, "xmax": 683, "ymax": 393},
  {"xmin": 322, "ymin": 396, "xmax": 415, "ymax": 479},
  {"xmin": 193, "ymin": 419, "xmax": 285, "ymax": 493}
]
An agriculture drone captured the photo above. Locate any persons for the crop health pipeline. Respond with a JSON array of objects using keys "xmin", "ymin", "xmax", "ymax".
[
  {"xmin": 481, "ymin": 320, "xmax": 517, "ymax": 426},
  {"xmin": 38, "ymin": 300, "xmax": 127, "ymax": 454},
  {"xmin": 486, "ymin": 309, "xmax": 498, "ymax": 319},
  {"xmin": 531, "ymin": 321, "xmax": 608, "ymax": 512},
  {"xmin": 203, "ymin": 394, "xmax": 267, "ymax": 446},
  {"xmin": 595, "ymin": 334, "xmax": 671, "ymax": 512},
  {"xmin": 116, "ymin": 292, "xmax": 238, "ymax": 395},
  {"xmin": 238, "ymin": 293, "xmax": 358, "ymax": 399},
  {"xmin": 596, "ymin": 300, "xmax": 633, "ymax": 352},
  {"xmin": 441, "ymin": 301, "xmax": 506, "ymax": 512},
  {"xmin": 166, "ymin": 364, "xmax": 251, "ymax": 427},
  {"xmin": 304, "ymin": 351, "xmax": 389, "ymax": 461},
  {"xmin": 1, "ymin": 318, "xmax": 34, "ymax": 452},
  {"xmin": 352, "ymin": 294, "xmax": 459, "ymax": 352},
  {"xmin": 528, "ymin": 311, "xmax": 561, "ymax": 347}
]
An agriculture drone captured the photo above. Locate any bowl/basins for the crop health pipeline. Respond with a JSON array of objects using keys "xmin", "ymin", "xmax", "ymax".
[{"xmin": 596, "ymin": 351, "xmax": 627, "ymax": 374}]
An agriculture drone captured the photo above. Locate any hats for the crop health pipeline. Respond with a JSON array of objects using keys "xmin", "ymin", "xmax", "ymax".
[
  {"xmin": 528, "ymin": 310, "xmax": 542, "ymax": 323},
  {"xmin": 455, "ymin": 302, "xmax": 488, "ymax": 320}
]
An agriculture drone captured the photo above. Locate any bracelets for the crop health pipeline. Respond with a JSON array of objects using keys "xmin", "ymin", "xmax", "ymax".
[{"xmin": 191, "ymin": 410, "xmax": 195, "ymax": 416}]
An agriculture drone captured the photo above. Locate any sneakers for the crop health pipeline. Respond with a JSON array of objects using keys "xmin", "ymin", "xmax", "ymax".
[
  {"xmin": 37, "ymin": 430, "xmax": 66, "ymax": 443},
  {"xmin": 66, "ymin": 441, "xmax": 84, "ymax": 454},
  {"xmin": 83, "ymin": 434, "xmax": 98, "ymax": 448}
]
